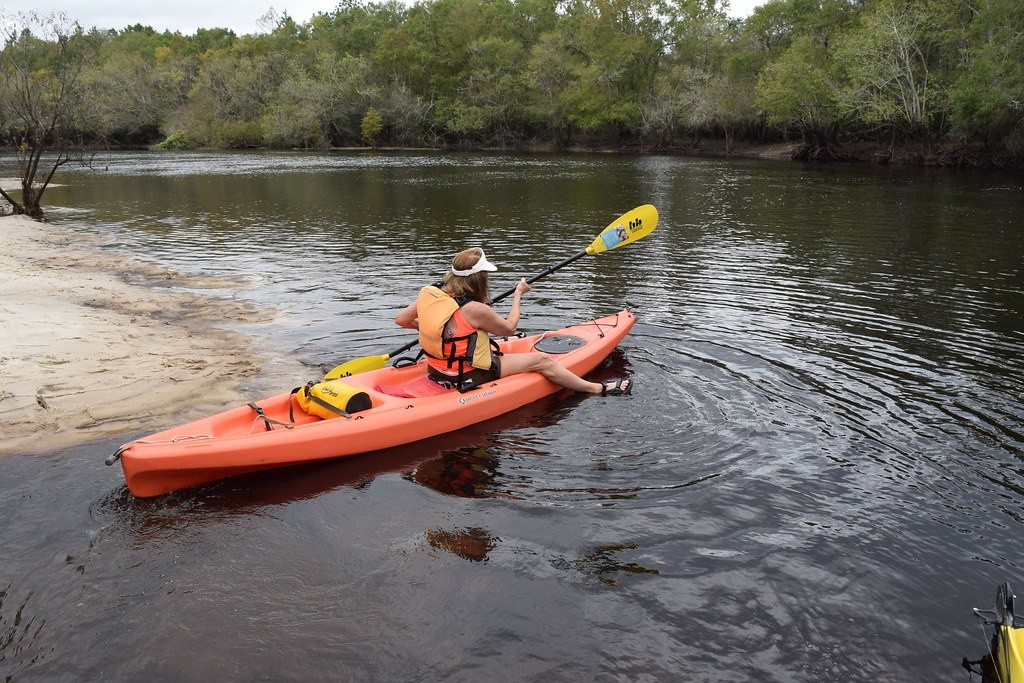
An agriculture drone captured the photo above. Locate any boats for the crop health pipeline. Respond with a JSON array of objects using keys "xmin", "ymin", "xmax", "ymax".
[{"xmin": 106, "ymin": 304, "xmax": 639, "ymax": 498}]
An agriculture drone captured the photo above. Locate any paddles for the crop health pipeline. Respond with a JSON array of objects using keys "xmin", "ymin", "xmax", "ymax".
[{"xmin": 322, "ymin": 202, "xmax": 660, "ymax": 382}]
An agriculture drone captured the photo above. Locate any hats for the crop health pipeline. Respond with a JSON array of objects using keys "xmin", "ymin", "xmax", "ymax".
[{"xmin": 452, "ymin": 247, "xmax": 497, "ymax": 276}]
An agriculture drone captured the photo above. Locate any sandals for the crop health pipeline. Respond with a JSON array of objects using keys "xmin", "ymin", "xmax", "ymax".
[{"xmin": 598, "ymin": 377, "xmax": 633, "ymax": 397}]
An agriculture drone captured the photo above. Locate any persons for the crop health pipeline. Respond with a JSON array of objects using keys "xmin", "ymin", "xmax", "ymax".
[{"xmin": 393, "ymin": 248, "xmax": 633, "ymax": 397}]
